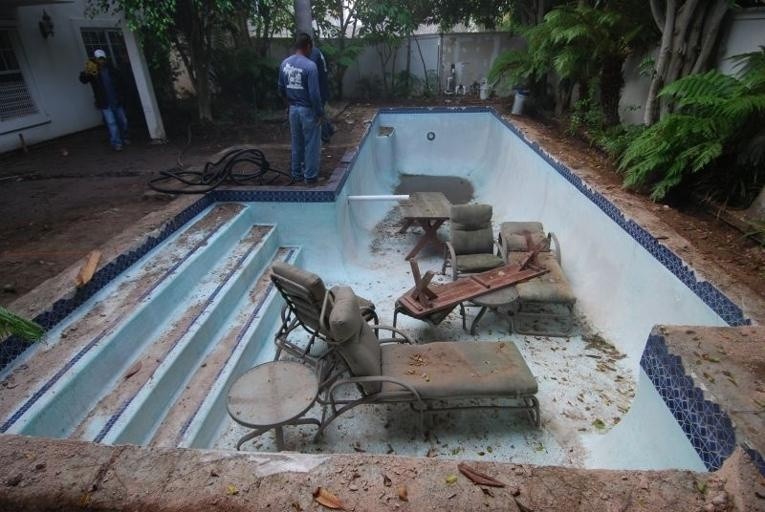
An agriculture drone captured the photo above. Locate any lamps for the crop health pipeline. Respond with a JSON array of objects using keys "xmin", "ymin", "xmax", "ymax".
[{"xmin": 37, "ymin": 7, "xmax": 56, "ymax": 40}]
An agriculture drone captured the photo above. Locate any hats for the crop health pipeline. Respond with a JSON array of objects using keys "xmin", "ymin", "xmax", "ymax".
[{"xmin": 94, "ymin": 49, "xmax": 107, "ymax": 58}]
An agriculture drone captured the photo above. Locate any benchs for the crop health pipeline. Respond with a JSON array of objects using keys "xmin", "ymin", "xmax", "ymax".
[{"xmin": 397, "ymin": 229, "xmax": 551, "ymax": 319}]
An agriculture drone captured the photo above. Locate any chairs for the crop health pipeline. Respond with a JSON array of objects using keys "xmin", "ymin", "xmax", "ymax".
[
  {"xmin": 267, "ymin": 260, "xmax": 380, "ymax": 395},
  {"xmin": 311, "ymin": 285, "xmax": 543, "ymax": 445},
  {"xmin": 441, "ymin": 202, "xmax": 504, "ymax": 281},
  {"xmin": 495, "ymin": 221, "xmax": 577, "ymax": 315}
]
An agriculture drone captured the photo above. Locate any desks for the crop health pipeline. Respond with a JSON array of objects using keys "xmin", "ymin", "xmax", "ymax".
[
  {"xmin": 389, "ymin": 289, "xmax": 466, "ymax": 343},
  {"xmin": 226, "ymin": 358, "xmax": 323, "ymax": 453},
  {"xmin": 393, "ymin": 191, "xmax": 454, "ymax": 261},
  {"xmin": 460, "ymin": 281, "xmax": 519, "ymax": 337}
]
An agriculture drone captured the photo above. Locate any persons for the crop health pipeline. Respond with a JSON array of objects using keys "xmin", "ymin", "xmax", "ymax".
[
  {"xmin": 307, "ymin": 48, "xmax": 337, "ymax": 142},
  {"xmin": 276, "ymin": 31, "xmax": 326, "ymax": 186},
  {"xmin": 79, "ymin": 49, "xmax": 132, "ymax": 153}
]
